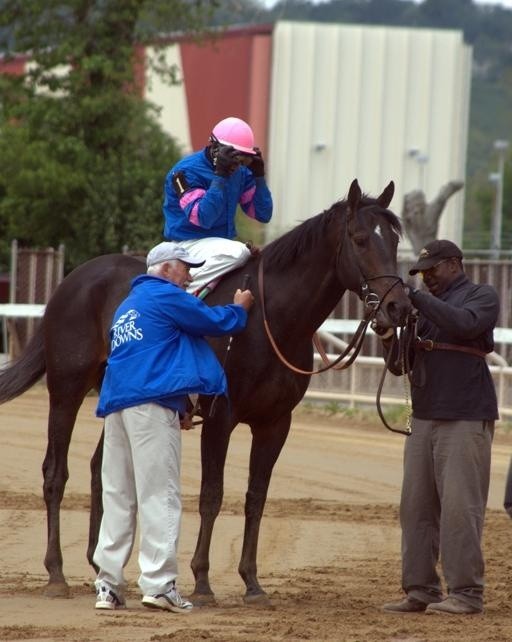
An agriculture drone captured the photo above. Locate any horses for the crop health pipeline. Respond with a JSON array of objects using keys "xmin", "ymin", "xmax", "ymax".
[{"xmin": 1, "ymin": 177, "xmax": 413, "ymax": 608}]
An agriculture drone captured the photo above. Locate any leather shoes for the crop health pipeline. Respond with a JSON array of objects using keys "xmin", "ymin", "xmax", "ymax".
[
  {"xmin": 425, "ymin": 593, "xmax": 483, "ymax": 617},
  {"xmin": 379, "ymin": 593, "xmax": 443, "ymax": 613}
]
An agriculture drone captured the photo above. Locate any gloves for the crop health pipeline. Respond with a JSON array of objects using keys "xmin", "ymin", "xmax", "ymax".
[
  {"xmin": 245, "ymin": 146, "xmax": 267, "ymax": 179},
  {"xmin": 213, "ymin": 145, "xmax": 243, "ymax": 180},
  {"xmin": 370, "ymin": 318, "xmax": 395, "ymax": 340},
  {"xmin": 402, "ymin": 283, "xmax": 422, "ymax": 302}
]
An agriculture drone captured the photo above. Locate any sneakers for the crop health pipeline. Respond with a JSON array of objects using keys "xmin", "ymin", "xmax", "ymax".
[
  {"xmin": 138, "ymin": 585, "xmax": 194, "ymax": 615},
  {"xmin": 93, "ymin": 578, "xmax": 129, "ymax": 612}
]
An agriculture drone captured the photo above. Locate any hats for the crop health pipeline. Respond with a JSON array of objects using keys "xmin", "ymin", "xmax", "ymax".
[
  {"xmin": 144, "ymin": 242, "xmax": 206, "ymax": 268},
  {"xmin": 408, "ymin": 240, "xmax": 463, "ymax": 276}
]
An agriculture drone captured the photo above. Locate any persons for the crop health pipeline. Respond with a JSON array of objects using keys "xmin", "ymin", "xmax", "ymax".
[
  {"xmin": 90, "ymin": 241, "xmax": 256, "ymax": 611},
  {"xmin": 369, "ymin": 238, "xmax": 499, "ymax": 618},
  {"xmin": 162, "ymin": 116, "xmax": 274, "ymax": 304}
]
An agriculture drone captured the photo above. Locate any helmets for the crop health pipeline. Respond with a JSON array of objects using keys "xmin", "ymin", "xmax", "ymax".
[{"xmin": 210, "ymin": 115, "xmax": 255, "ymax": 154}]
